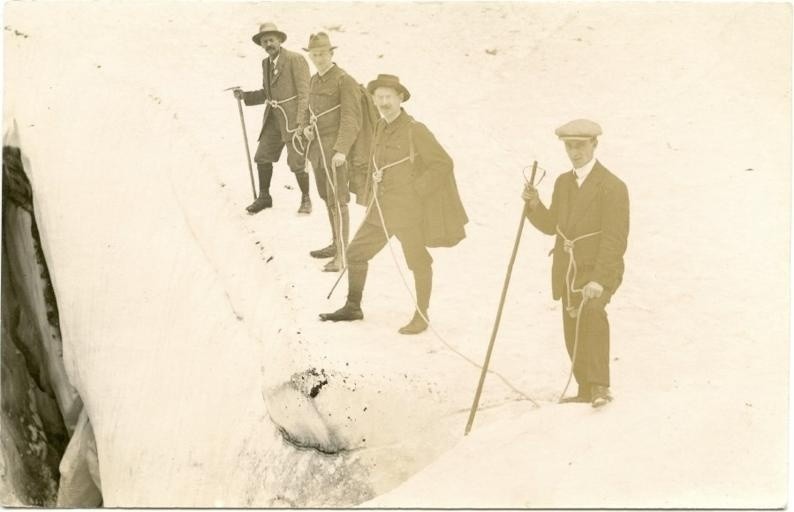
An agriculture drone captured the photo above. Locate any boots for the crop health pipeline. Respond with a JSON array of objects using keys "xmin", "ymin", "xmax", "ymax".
[
  {"xmin": 310, "ymin": 209, "xmax": 349, "ymax": 273},
  {"xmin": 397, "ymin": 270, "xmax": 433, "ymax": 336},
  {"xmin": 559, "ymin": 383, "xmax": 592, "ymax": 404},
  {"xmin": 296, "ymin": 173, "xmax": 312, "ymax": 214},
  {"xmin": 589, "ymin": 383, "xmax": 609, "ymax": 408},
  {"xmin": 319, "ymin": 268, "xmax": 368, "ymax": 322},
  {"xmin": 246, "ymin": 166, "xmax": 273, "ymax": 214}
]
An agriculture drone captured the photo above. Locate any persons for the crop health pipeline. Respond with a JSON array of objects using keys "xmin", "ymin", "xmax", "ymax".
[
  {"xmin": 319, "ymin": 74, "xmax": 468, "ymax": 335},
  {"xmin": 309, "ymin": 31, "xmax": 363, "ymax": 273},
  {"xmin": 520, "ymin": 119, "xmax": 629, "ymax": 408},
  {"xmin": 231, "ymin": 22, "xmax": 312, "ymax": 215}
]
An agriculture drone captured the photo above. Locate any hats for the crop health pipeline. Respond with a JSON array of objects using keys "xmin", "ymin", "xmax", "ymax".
[
  {"xmin": 554, "ymin": 118, "xmax": 604, "ymax": 141},
  {"xmin": 252, "ymin": 22, "xmax": 288, "ymax": 46},
  {"xmin": 366, "ymin": 73, "xmax": 410, "ymax": 103},
  {"xmin": 302, "ymin": 31, "xmax": 338, "ymax": 52}
]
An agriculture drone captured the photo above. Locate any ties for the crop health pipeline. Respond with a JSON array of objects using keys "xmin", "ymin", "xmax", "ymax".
[
  {"xmin": 269, "ymin": 62, "xmax": 274, "ymax": 86},
  {"xmin": 565, "ymin": 171, "xmax": 582, "ymax": 220}
]
